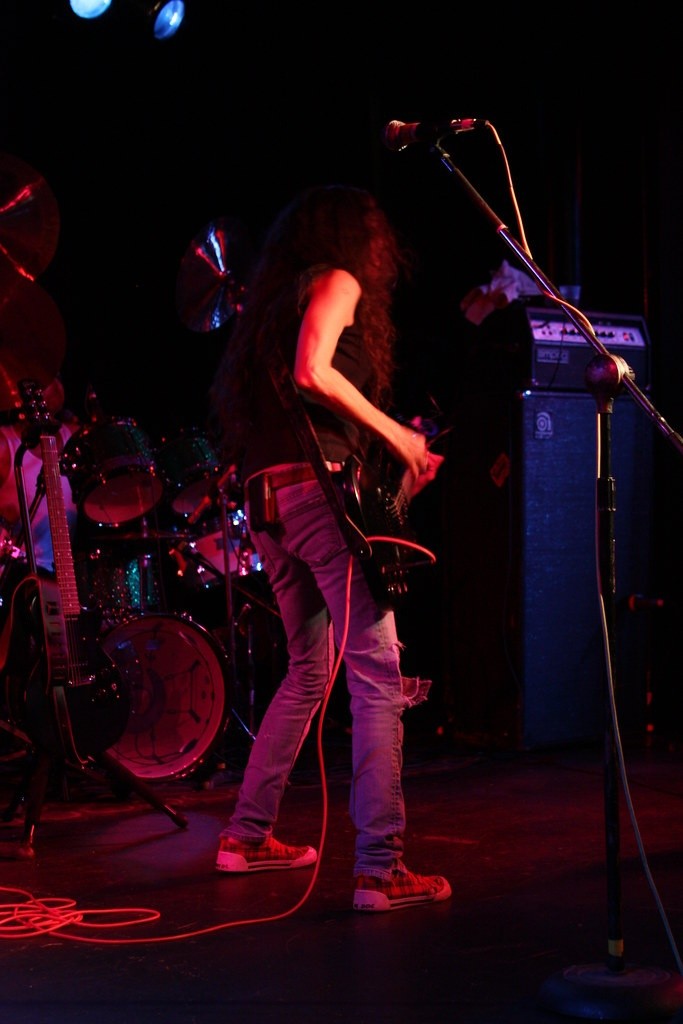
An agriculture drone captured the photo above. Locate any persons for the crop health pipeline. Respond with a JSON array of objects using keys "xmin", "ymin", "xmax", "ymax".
[
  {"xmin": 209, "ymin": 183, "xmax": 454, "ymax": 914},
  {"xmin": 0, "ymin": 374, "xmax": 82, "ymax": 572}
]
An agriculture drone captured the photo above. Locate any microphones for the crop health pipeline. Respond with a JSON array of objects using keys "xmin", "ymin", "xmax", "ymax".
[
  {"xmin": 628, "ymin": 594, "xmax": 666, "ymax": 610},
  {"xmin": 238, "ymin": 605, "xmax": 252, "ymax": 636},
  {"xmin": 188, "ymin": 465, "xmax": 238, "ymax": 524},
  {"xmin": 87, "ymin": 384, "xmax": 103, "ymax": 417},
  {"xmin": 384, "ymin": 118, "xmax": 489, "ymax": 151},
  {"xmin": 170, "ymin": 538, "xmax": 205, "ymax": 559}
]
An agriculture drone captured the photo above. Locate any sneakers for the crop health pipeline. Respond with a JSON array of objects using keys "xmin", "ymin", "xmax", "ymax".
[
  {"xmin": 216, "ymin": 838, "xmax": 318, "ymax": 873},
  {"xmin": 354, "ymin": 861, "xmax": 451, "ymax": 911}
]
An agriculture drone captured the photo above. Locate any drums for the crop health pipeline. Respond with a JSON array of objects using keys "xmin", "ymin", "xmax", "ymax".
[
  {"xmin": 97, "ymin": 609, "xmax": 235, "ymax": 780},
  {"xmin": 156, "ymin": 425, "xmax": 230, "ymax": 515},
  {"xmin": 61, "ymin": 418, "xmax": 166, "ymax": 529}
]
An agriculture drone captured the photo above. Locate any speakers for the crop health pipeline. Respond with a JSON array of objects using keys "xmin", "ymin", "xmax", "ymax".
[{"xmin": 438, "ymin": 389, "xmax": 654, "ymax": 750}]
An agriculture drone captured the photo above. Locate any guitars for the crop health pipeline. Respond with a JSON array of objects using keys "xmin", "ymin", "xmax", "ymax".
[
  {"xmin": 331, "ymin": 399, "xmax": 463, "ymax": 604},
  {"xmin": 16, "ymin": 375, "xmax": 137, "ymax": 760}
]
